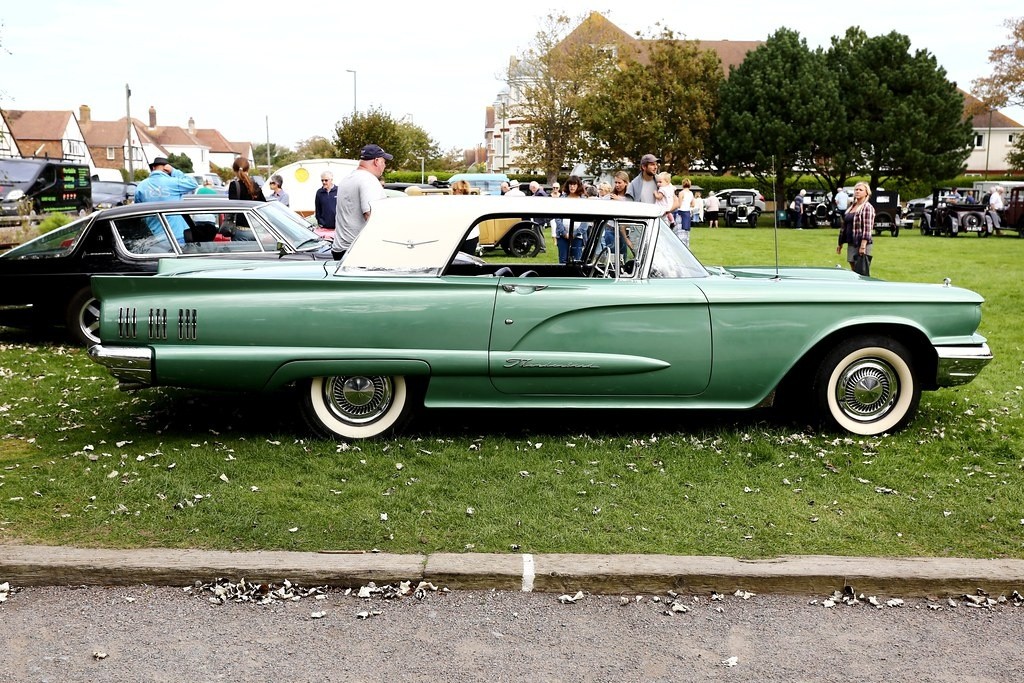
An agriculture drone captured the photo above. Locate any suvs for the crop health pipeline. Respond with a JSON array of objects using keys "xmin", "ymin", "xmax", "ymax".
[
  {"xmin": 801, "ymin": 192, "xmax": 842, "ymax": 229},
  {"xmin": 726, "ymin": 191, "xmax": 758, "ymax": 229},
  {"xmin": 843, "ymin": 188, "xmax": 993, "ymax": 237},
  {"xmin": 997, "ymin": 185, "xmax": 1024, "ymax": 238}
]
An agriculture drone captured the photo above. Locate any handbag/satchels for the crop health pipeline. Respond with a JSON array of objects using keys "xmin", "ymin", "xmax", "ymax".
[
  {"xmin": 853, "ymin": 252, "xmax": 873, "ymax": 277},
  {"xmin": 218, "ymin": 219, "xmax": 236, "ymax": 237}
]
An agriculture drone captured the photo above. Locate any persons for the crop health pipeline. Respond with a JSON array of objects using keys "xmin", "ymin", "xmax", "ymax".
[
  {"xmin": 315, "ymin": 171, "xmax": 340, "ymax": 229},
  {"xmin": 331, "ymin": 145, "xmax": 394, "ymax": 262},
  {"xmin": 132, "ymin": 157, "xmax": 199, "ymax": 247},
  {"xmin": 268, "ymin": 175, "xmax": 288, "ymax": 208},
  {"xmin": 947, "ymin": 186, "xmax": 1007, "ymax": 237},
  {"xmin": 795, "ymin": 189, "xmax": 807, "ymax": 231},
  {"xmin": 835, "ymin": 188, "xmax": 850, "ymax": 220},
  {"xmin": 227, "ymin": 158, "xmax": 266, "ymax": 243},
  {"xmin": 836, "ymin": 181, "xmax": 876, "ymax": 271},
  {"xmin": 377, "ymin": 153, "xmax": 720, "ymax": 264}
]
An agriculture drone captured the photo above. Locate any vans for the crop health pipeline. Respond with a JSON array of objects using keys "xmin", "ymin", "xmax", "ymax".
[{"xmin": 0, "ymin": 159, "xmax": 91, "ymax": 226}]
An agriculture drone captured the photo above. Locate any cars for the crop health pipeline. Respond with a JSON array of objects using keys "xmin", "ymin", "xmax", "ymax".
[
  {"xmin": 383, "ymin": 183, "xmax": 440, "ymax": 192},
  {"xmin": 0, "ymin": 198, "xmax": 493, "ymax": 347},
  {"xmin": 186, "ymin": 173, "xmax": 265, "ymax": 192},
  {"xmin": 476, "ymin": 218, "xmax": 542, "ymax": 258},
  {"xmin": 715, "ymin": 188, "xmax": 766, "ymax": 216},
  {"xmin": 87, "ymin": 193, "xmax": 995, "ymax": 438},
  {"xmin": 91, "ymin": 181, "xmax": 138, "ymax": 210}
]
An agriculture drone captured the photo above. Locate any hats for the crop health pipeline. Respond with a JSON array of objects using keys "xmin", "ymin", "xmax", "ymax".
[
  {"xmin": 359, "ymin": 144, "xmax": 393, "ymax": 160},
  {"xmin": 507, "ymin": 180, "xmax": 522, "ymax": 188},
  {"xmin": 203, "ymin": 175, "xmax": 212, "ymax": 184},
  {"xmin": 641, "ymin": 154, "xmax": 661, "ymax": 164},
  {"xmin": 149, "ymin": 157, "xmax": 169, "ymax": 168}
]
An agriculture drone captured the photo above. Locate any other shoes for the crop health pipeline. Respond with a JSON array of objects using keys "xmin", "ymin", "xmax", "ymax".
[{"xmin": 669, "ymin": 222, "xmax": 676, "ymax": 230}]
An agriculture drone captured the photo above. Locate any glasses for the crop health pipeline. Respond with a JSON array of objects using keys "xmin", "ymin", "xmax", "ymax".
[
  {"xmin": 321, "ymin": 178, "xmax": 331, "ymax": 182},
  {"xmin": 270, "ymin": 182, "xmax": 277, "ymax": 185},
  {"xmin": 553, "ymin": 187, "xmax": 559, "ymax": 189}
]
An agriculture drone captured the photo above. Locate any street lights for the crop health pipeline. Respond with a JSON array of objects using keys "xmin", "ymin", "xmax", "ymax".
[
  {"xmin": 345, "ymin": 70, "xmax": 356, "ymax": 115},
  {"xmin": 417, "ymin": 157, "xmax": 424, "ymax": 184}
]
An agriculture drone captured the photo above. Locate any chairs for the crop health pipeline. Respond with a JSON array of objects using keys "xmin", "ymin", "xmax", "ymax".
[{"xmin": 493, "ymin": 267, "xmax": 539, "ymax": 279}]
